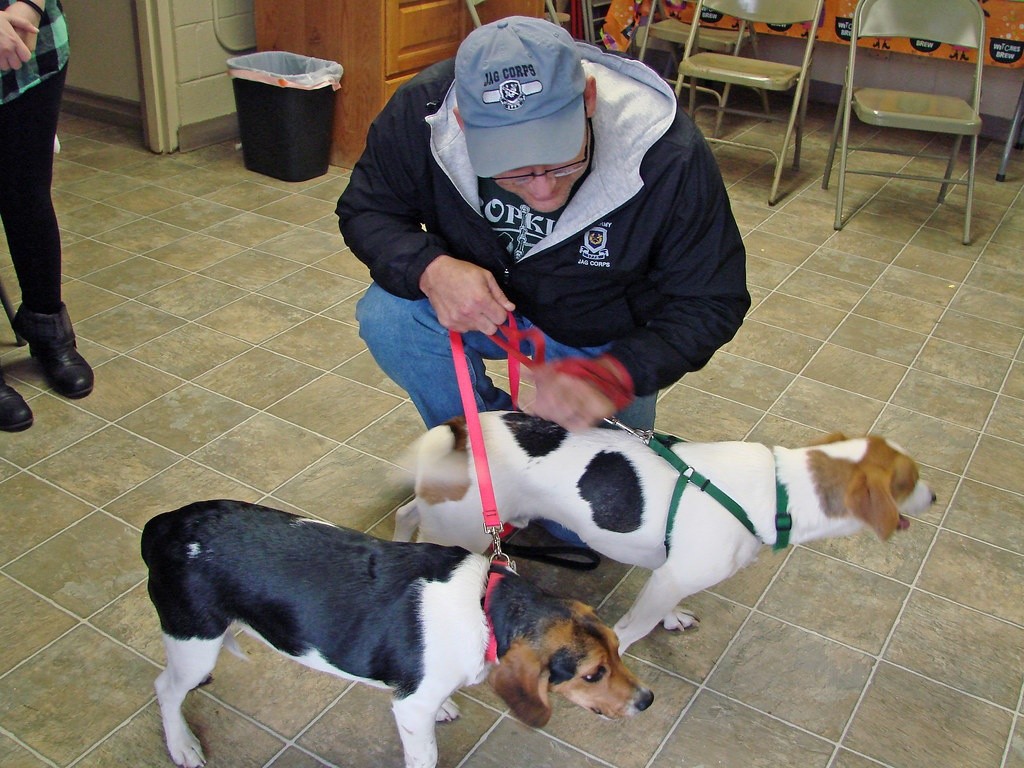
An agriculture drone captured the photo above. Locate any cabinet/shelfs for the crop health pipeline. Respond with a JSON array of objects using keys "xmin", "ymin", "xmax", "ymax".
[{"xmin": 254, "ymin": 0, "xmax": 545, "ymax": 172}]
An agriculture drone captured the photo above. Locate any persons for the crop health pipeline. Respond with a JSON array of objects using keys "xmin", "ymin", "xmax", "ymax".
[
  {"xmin": 334, "ymin": 16, "xmax": 752, "ymax": 549},
  {"xmin": 0, "ymin": 0, "xmax": 95, "ymax": 430}
]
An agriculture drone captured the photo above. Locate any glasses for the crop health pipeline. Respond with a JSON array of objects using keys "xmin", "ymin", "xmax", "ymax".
[{"xmin": 491, "ymin": 94, "xmax": 592, "ymax": 188}]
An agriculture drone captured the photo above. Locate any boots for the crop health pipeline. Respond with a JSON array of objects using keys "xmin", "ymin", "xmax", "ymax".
[
  {"xmin": 13, "ymin": 298, "xmax": 94, "ymax": 397},
  {"xmin": 0, "ymin": 378, "xmax": 32, "ymax": 430}
]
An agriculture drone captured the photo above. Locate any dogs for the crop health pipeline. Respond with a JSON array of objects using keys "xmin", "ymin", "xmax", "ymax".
[
  {"xmin": 389, "ymin": 410, "xmax": 937, "ymax": 659},
  {"xmin": 141, "ymin": 498, "xmax": 655, "ymax": 768}
]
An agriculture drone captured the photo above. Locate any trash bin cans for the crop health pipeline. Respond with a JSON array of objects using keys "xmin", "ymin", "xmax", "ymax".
[{"xmin": 225, "ymin": 51, "xmax": 344, "ymax": 184}]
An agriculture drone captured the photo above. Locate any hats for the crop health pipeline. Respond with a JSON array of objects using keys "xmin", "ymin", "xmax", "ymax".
[{"xmin": 455, "ymin": 14, "xmax": 587, "ymax": 177}]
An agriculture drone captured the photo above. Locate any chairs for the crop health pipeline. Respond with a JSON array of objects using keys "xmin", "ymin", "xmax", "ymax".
[
  {"xmin": 674, "ymin": 0, "xmax": 823, "ymax": 206},
  {"xmin": 463, "ymin": 0, "xmax": 620, "ymax": 52},
  {"xmin": 638, "ymin": 0, "xmax": 771, "ymax": 144},
  {"xmin": 822, "ymin": 0, "xmax": 986, "ymax": 243}
]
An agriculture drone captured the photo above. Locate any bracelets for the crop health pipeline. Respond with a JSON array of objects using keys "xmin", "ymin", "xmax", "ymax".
[{"xmin": 17, "ymin": 0, "xmax": 44, "ymax": 19}]
